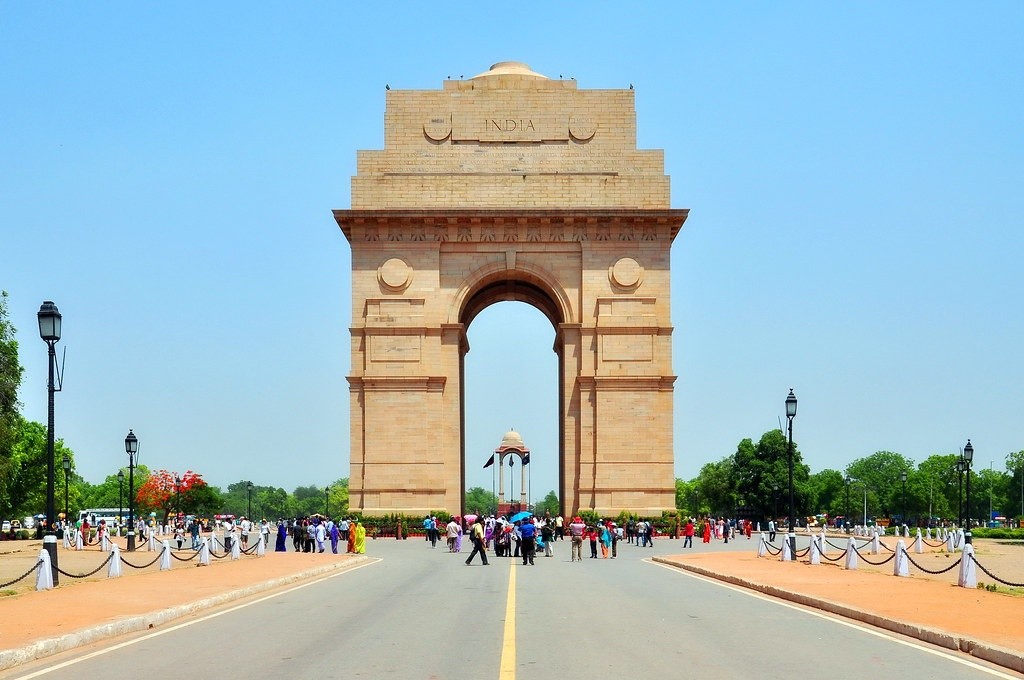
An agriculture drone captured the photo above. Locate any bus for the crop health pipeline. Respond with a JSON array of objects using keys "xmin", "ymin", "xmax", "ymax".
[{"xmin": 78, "ymin": 507, "xmax": 135, "ymax": 531}]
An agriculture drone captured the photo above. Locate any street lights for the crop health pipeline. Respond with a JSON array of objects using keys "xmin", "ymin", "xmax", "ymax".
[
  {"xmin": 845, "ymin": 476, "xmax": 850, "ymax": 533},
  {"xmin": 175, "ymin": 475, "xmax": 180, "ymax": 523},
  {"xmin": 117, "ymin": 470, "xmax": 125, "ymax": 537},
  {"xmin": 36, "ymin": 299, "xmax": 62, "ymax": 586},
  {"xmin": 990, "ymin": 460, "xmax": 995, "ymax": 521},
  {"xmin": 125, "ymin": 428, "xmax": 138, "ymax": 532},
  {"xmin": 62, "ymin": 454, "xmax": 71, "ymax": 527},
  {"xmin": 772, "ymin": 483, "xmax": 779, "ymax": 532},
  {"xmin": 785, "ymin": 388, "xmax": 798, "ymax": 561},
  {"xmin": 964, "ymin": 439, "xmax": 974, "ymax": 545},
  {"xmin": 247, "ymin": 481, "xmax": 253, "ymax": 521},
  {"xmin": 325, "ymin": 486, "xmax": 330, "ymax": 520},
  {"xmin": 694, "ymin": 485, "xmax": 699, "ymax": 519},
  {"xmin": 902, "ymin": 468, "xmax": 908, "ymax": 536},
  {"xmin": 957, "ymin": 454, "xmax": 967, "ymax": 528}
]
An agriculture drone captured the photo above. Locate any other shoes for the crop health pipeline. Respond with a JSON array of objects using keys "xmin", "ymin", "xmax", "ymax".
[
  {"xmin": 611, "ymin": 556, "xmax": 617, "ymax": 559},
  {"xmin": 465, "ymin": 561, "xmax": 471, "ymax": 565},
  {"xmin": 145, "ymin": 538, "xmax": 147, "ymax": 542},
  {"xmin": 295, "ymin": 548, "xmax": 315, "ymax": 553},
  {"xmin": 590, "ymin": 555, "xmax": 598, "ymax": 558},
  {"xmin": 138, "ymin": 540, "xmax": 142, "ymax": 542},
  {"xmin": 483, "ymin": 563, "xmax": 490, "ymax": 565},
  {"xmin": 318, "ymin": 549, "xmax": 324, "ymax": 553}
]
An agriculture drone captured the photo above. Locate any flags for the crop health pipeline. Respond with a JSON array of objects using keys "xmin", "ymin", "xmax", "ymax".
[
  {"xmin": 509, "ymin": 456, "xmax": 514, "ymax": 467},
  {"xmin": 483, "ymin": 454, "xmax": 494, "ymax": 468},
  {"xmin": 522, "ymin": 454, "xmax": 529, "ymax": 466}
]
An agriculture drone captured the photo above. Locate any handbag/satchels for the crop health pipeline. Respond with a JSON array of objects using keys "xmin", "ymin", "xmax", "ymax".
[{"xmin": 174, "ymin": 533, "xmax": 177, "ymax": 539}]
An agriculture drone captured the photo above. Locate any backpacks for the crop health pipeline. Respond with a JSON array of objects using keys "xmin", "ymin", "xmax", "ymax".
[
  {"xmin": 644, "ymin": 522, "xmax": 653, "ymax": 535},
  {"xmin": 628, "ymin": 521, "xmax": 635, "ymax": 534}
]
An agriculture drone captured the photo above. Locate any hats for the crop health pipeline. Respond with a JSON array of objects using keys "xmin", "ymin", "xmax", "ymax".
[
  {"xmin": 262, "ymin": 518, "xmax": 267, "ymax": 522},
  {"xmin": 599, "ymin": 518, "xmax": 604, "ymax": 522},
  {"xmin": 573, "ymin": 516, "xmax": 582, "ymax": 523},
  {"xmin": 489, "ymin": 515, "xmax": 494, "ymax": 518},
  {"xmin": 431, "ymin": 516, "xmax": 436, "ymax": 521},
  {"xmin": 610, "ymin": 522, "xmax": 617, "ymax": 527}
]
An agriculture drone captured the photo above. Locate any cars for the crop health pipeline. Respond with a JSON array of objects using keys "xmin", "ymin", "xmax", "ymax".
[{"xmin": 1, "ymin": 516, "xmax": 35, "ymax": 531}]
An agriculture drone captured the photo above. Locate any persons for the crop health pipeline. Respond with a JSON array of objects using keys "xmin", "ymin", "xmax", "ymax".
[
  {"xmin": 346, "ymin": 522, "xmax": 356, "ymax": 552},
  {"xmin": 261, "ymin": 520, "xmax": 270, "ymax": 548},
  {"xmin": 769, "ymin": 518, "xmax": 776, "ymax": 542},
  {"xmin": 684, "ymin": 519, "xmax": 694, "ymax": 548},
  {"xmin": 339, "ymin": 515, "xmax": 358, "ymax": 541},
  {"xmin": 224, "ymin": 516, "xmax": 250, "ymax": 551},
  {"xmin": 423, "ymin": 514, "xmax": 442, "ymax": 548},
  {"xmin": 173, "ymin": 519, "xmax": 211, "ymax": 551},
  {"xmin": 483, "ymin": 513, "xmax": 564, "ymax": 565},
  {"xmin": 703, "ymin": 516, "xmax": 752, "ymax": 543},
  {"xmin": 113, "ymin": 517, "xmax": 147, "ymax": 542},
  {"xmin": 275, "ymin": 514, "xmax": 334, "ymax": 553},
  {"xmin": 32, "ymin": 518, "xmax": 107, "ymax": 546},
  {"xmin": 355, "ymin": 523, "xmax": 365, "ymax": 554},
  {"xmin": 465, "ymin": 516, "xmax": 490, "ymax": 565},
  {"xmin": 568, "ymin": 517, "xmax": 624, "ymax": 562},
  {"xmin": 626, "ymin": 516, "xmax": 653, "ymax": 547},
  {"xmin": 328, "ymin": 525, "xmax": 343, "ymax": 553},
  {"xmin": 446, "ymin": 517, "xmax": 463, "ymax": 552}
]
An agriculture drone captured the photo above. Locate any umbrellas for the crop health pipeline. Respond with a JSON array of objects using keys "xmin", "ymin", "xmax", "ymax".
[
  {"xmin": 34, "ymin": 514, "xmax": 46, "ymax": 521},
  {"xmin": 509, "ymin": 511, "xmax": 533, "ymax": 523}
]
